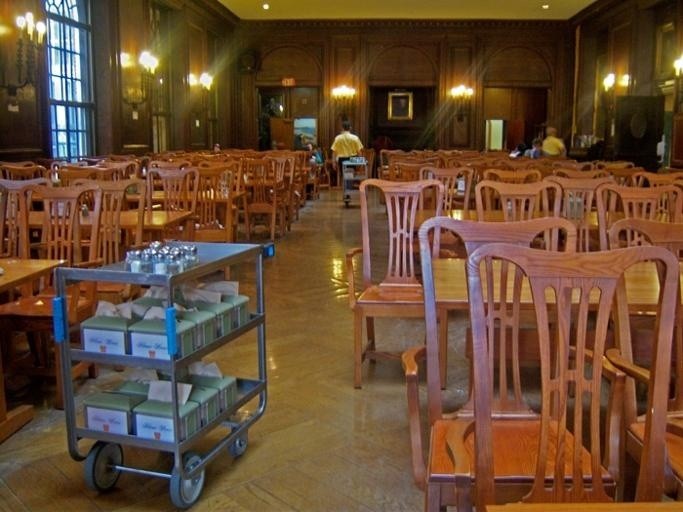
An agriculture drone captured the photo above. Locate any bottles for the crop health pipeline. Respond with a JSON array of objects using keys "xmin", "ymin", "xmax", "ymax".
[
  {"xmin": 124, "ymin": 240, "xmax": 201, "ymax": 277},
  {"xmin": 81, "ymin": 204, "xmax": 90, "ymax": 225}
]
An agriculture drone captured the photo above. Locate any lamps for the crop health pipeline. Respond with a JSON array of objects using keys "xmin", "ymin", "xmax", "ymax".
[
  {"xmin": 122, "ymin": 51, "xmax": 158, "ymax": 111},
  {"xmin": 189, "ymin": 74, "xmax": 213, "ymax": 115},
  {"xmin": 0, "ymin": 11, "xmax": 46, "ymax": 97},
  {"xmin": 332, "ymin": 84, "xmax": 355, "ymax": 115},
  {"xmin": 603, "ymin": 72, "xmax": 616, "ymax": 96},
  {"xmin": 451, "ymin": 85, "xmax": 473, "ymax": 117},
  {"xmin": 672, "ymin": 55, "xmax": 683, "ymax": 77}
]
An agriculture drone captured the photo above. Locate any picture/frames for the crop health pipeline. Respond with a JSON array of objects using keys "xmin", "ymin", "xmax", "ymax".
[
  {"xmin": 387, "ymin": 92, "xmax": 413, "ymax": 120},
  {"xmin": 291, "ymin": 115, "xmax": 320, "ymax": 151}
]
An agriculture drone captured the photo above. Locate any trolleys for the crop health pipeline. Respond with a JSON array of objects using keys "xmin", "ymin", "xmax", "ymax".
[
  {"xmin": 342, "ymin": 156, "xmax": 369, "ymax": 208},
  {"xmin": 51, "ymin": 243, "xmax": 277, "ymax": 503}
]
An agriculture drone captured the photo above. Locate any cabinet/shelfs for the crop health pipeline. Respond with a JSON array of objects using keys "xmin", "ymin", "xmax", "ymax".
[{"xmin": 615, "ymin": 95, "xmax": 666, "ymax": 173}]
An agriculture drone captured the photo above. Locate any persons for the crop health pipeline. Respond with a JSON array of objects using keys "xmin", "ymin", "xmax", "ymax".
[
  {"xmin": 328, "ymin": 120, "xmax": 365, "ymax": 208},
  {"xmin": 515, "ymin": 125, "xmax": 569, "ymax": 160},
  {"xmin": 304, "ymin": 140, "xmax": 325, "ymax": 183}
]
